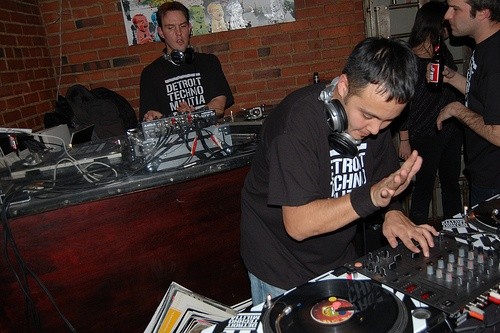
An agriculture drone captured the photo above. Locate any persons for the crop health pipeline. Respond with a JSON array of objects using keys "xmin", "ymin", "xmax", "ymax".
[
  {"xmin": 140, "ymin": 1, "xmax": 234, "ymax": 122},
  {"xmin": 399, "ymin": 0, "xmax": 500, "ymax": 224},
  {"xmin": 240, "ymin": 38, "xmax": 438, "ymax": 307}
]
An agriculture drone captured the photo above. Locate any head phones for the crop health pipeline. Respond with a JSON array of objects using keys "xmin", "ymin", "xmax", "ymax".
[
  {"xmin": 468, "ymin": 201, "xmax": 500, "ymax": 234},
  {"xmin": 162, "ymin": 45, "xmax": 195, "ymax": 67},
  {"xmin": 316, "ymin": 74, "xmax": 363, "ymax": 158}
]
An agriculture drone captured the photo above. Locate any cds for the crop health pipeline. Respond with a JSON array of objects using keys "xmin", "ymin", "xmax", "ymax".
[{"xmin": 262, "ymin": 278, "xmax": 408, "ymax": 333}]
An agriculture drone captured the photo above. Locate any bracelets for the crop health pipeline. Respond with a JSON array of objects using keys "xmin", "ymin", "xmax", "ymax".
[
  {"xmin": 380, "ymin": 198, "xmax": 407, "ymax": 218},
  {"xmin": 351, "ymin": 181, "xmax": 380, "ymax": 218}
]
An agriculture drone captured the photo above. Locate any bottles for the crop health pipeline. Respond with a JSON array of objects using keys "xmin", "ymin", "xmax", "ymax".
[
  {"xmin": 428, "ymin": 30, "xmax": 443, "ymax": 88},
  {"xmin": 313, "ymin": 72, "xmax": 320, "ymax": 84}
]
